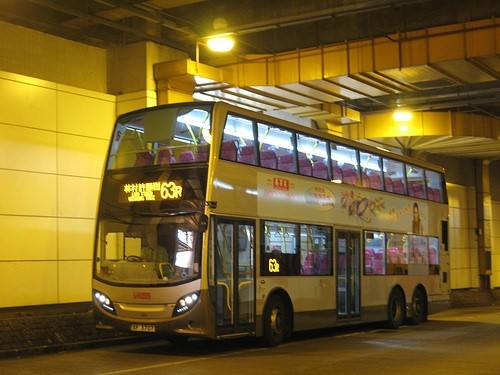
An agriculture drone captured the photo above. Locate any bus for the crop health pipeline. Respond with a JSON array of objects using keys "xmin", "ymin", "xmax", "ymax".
[{"xmin": 90, "ymin": 99, "xmax": 453, "ymax": 348}]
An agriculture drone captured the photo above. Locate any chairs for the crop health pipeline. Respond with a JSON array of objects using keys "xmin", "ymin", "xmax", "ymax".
[
  {"xmin": 132, "ymin": 140, "xmax": 440, "ymax": 203},
  {"xmin": 303, "ymin": 247, "xmax": 438, "ymax": 275}
]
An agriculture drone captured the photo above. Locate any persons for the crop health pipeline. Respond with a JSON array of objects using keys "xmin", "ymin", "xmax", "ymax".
[
  {"xmin": 412, "ymin": 203, "xmax": 423, "ymax": 234},
  {"xmin": 138, "ymin": 231, "xmax": 168, "ymax": 262}
]
